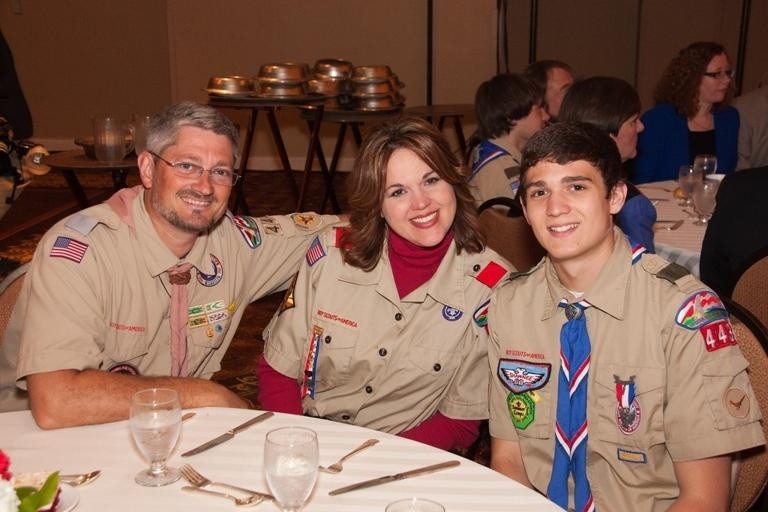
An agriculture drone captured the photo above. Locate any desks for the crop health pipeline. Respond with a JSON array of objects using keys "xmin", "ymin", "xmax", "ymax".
[
  {"xmin": 39, "ymin": 147, "xmax": 143, "ymax": 213},
  {"xmin": 207, "ymin": 91, "xmax": 342, "ymax": 217},
  {"xmin": 299, "ymin": 108, "xmax": 404, "ymax": 215},
  {"xmin": 404, "ymin": 102, "xmax": 477, "ymax": 162}
]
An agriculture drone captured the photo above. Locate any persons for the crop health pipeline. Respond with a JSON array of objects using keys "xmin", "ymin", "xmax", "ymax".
[
  {"xmin": 0, "ymin": 34, "xmax": 34, "ymax": 178},
  {"xmin": 258, "ymin": 113, "xmax": 519, "ymax": 450},
  {"xmin": 0, "ymin": 101, "xmax": 351, "ymax": 430},
  {"xmin": 486, "ymin": 114, "xmax": 766, "ymax": 512}
]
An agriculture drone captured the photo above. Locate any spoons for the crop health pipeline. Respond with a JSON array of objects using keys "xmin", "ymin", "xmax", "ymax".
[
  {"xmin": 53, "ymin": 471, "xmax": 103, "ymax": 487},
  {"xmin": 182, "ymin": 483, "xmax": 263, "ymax": 508}
]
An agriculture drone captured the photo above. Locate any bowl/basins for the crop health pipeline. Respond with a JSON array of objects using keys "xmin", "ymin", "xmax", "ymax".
[
  {"xmin": 200, "ymin": 54, "xmax": 407, "ymax": 115},
  {"xmin": 74, "ymin": 137, "xmax": 136, "ymax": 161}
]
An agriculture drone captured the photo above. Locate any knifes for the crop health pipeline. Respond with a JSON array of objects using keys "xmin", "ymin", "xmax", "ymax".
[
  {"xmin": 648, "ymin": 198, "xmax": 670, "ymax": 202},
  {"xmin": 654, "ymin": 219, "xmax": 678, "ymax": 224},
  {"xmin": 327, "ymin": 459, "xmax": 460, "ymax": 496},
  {"xmin": 178, "ymin": 412, "xmax": 275, "ymax": 458}
]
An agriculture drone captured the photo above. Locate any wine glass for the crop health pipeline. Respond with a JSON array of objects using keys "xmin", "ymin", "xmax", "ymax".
[
  {"xmin": 677, "ymin": 165, "xmax": 704, "ymax": 208},
  {"xmin": 690, "ymin": 179, "xmax": 722, "ymax": 227},
  {"xmin": 127, "ymin": 387, "xmax": 182, "ymax": 488},
  {"xmin": 264, "ymin": 426, "xmax": 321, "ymax": 512}
]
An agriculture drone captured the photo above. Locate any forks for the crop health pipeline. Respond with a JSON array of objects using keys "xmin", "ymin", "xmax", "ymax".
[
  {"xmin": 653, "ymin": 219, "xmax": 684, "ymax": 232},
  {"xmin": 318, "ymin": 438, "xmax": 378, "ymax": 474},
  {"xmin": 179, "ymin": 459, "xmax": 275, "ymax": 501}
]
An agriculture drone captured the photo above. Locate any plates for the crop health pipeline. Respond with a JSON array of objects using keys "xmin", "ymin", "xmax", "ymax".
[{"xmin": 0, "ymin": 476, "xmax": 81, "ymax": 512}]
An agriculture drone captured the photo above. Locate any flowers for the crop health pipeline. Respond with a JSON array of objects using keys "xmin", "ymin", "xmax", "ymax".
[{"xmin": 1, "ymin": 448, "xmax": 60, "ymax": 512}]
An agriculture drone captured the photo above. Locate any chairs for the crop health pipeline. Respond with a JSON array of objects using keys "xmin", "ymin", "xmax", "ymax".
[
  {"xmin": 725, "ymin": 254, "xmax": 767, "ymax": 329},
  {"xmin": 0, "ymin": 260, "xmax": 32, "ymax": 349},
  {"xmin": 717, "ymin": 295, "xmax": 768, "ymax": 511},
  {"xmin": 476, "ymin": 195, "xmax": 549, "ymax": 273}
]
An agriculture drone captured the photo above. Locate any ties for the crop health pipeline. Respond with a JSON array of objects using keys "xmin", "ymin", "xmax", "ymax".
[
  {"xmin": 166, "ymin": 263, "xmax": 194, "ymax": 376},
  {"xmin": 543, "ymin": 298, "xmax": 599, "ymax": 512}
]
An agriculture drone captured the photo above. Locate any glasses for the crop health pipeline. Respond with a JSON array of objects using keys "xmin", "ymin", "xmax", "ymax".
[
  {"xmin": 701, "ymin": 68, "xmax": 737, "ymax": 80},
  {"xmin": 143, "ymin": 147, "xmax": 246, "ymax": 189}
]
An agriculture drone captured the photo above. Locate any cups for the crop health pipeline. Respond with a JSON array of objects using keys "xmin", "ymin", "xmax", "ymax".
[
  {"xmin": 385, "ymin": 496, "xmax": 445, "ymax": 512},
  {"xmin": 131, "ymin": 112, "xmax": 159, "ymax": 158},
  {"xmin": 694, "ymin": 153, "xmax": 719, "ymax": 181},
  {"xmin": 92, "ymin": 115, "xmax": 136, "ymax": 165}
]
